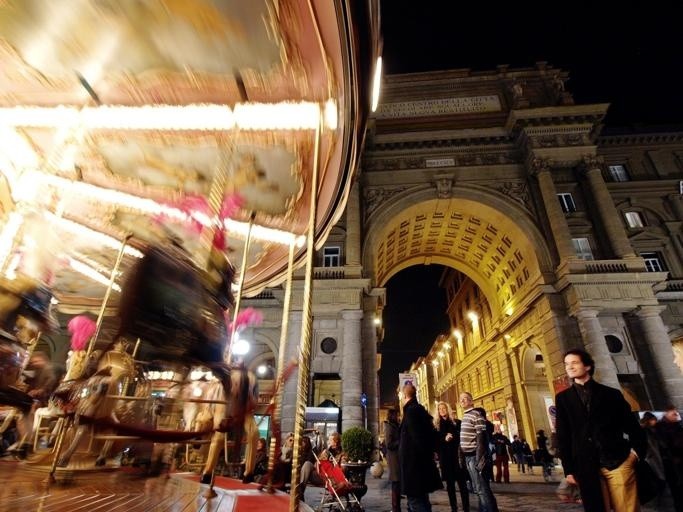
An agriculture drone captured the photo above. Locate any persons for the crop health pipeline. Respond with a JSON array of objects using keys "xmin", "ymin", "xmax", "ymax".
[
  {"xmin": 240, "ymin": 431, "xmax": 343, "ymax": 503},
  {"xmin": 398, "ymin": 383, "xmax": 445, "ymax": 512},
  {"xmin": 630, "ymin": 410, "xmax": 683, "ymax": 511},
  {"xmin": 670, "ymin": 335, "xmax": 683, "ymax": 374},
  {"xmin": 554, "ymin": 349, "xmax": 645, "ymax": 512},
  {"xmin": 432, "ymin": 408, "xmax": 552, "ymax": 486},
  {"xmin": 433, "ymin": 403, "xmax": 471, "ymax": 512},
  {"xmin": 459, "ymin": 392, "xmax": 498, "ymax": 512},
  {"xmin": 384, "ymin": 409, "xmax": 406, "ymax": 512}
]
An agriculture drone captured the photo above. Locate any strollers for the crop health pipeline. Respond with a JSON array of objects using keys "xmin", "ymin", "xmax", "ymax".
[{"xmin": 308, "ymin": 439, "xmax": 366, "ymax": 510}]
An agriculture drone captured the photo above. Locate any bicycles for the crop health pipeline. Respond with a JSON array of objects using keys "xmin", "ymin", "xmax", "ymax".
[{"xmin": 542, "ymin": 464, "xmax": 556, "ymax": 481}]
